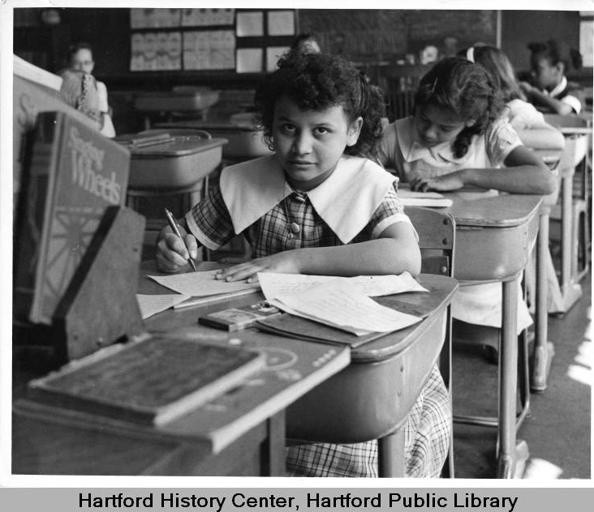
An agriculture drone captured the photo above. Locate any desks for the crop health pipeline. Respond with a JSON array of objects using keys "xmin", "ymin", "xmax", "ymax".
[{"xmin": 12, "ymin": 87, "xmax": 592, "ymax": 478}]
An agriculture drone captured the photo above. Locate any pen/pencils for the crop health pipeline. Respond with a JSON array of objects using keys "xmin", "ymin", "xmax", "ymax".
[
  {"xmin": 376, "ymin": 158, "xmax": 385, "ymax": 169},
  {"xmin": 164, "ymin": 208, "xmax": 196, "ymax": 272}
]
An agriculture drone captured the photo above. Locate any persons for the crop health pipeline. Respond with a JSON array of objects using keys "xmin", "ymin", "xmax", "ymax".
[
  {"xmin": 157, "ymin": 54, "xmax": 452, "ymax": 477},
  {"xmin": 517, "ymin": 40, "xmax": 586, "ymax": 118},
  {"xmin": 291, "ymin": 28, "xmax": 329, "ymax": 57},
  {"xmin": 457, "ymin": 44, "xmax": 567, "ymax": 316},
  {"xmin": 358, "ymin": 59, "xmax": 535, "ymax": 334}
]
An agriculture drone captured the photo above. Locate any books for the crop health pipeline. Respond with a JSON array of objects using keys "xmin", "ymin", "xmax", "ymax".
[
  {"xmin": 11, "ymin": 329, "xmax": 351, "ymax": 454},
  {"xmin": 13, "ymin": 49, "xmax": 174, "ymax": 326},
  {"xmin": 253, "ymin": 296, "xmax": 431, "ymax": 349},
  {"xmin": 24, "ymin": 336, "xmax": 266, "ymax": 425}
]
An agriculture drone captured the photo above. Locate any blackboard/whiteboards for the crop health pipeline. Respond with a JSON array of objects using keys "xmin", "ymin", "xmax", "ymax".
[{"xmin": 294, "ymin": 9, "xmax": 502, "ymax": 68}]
